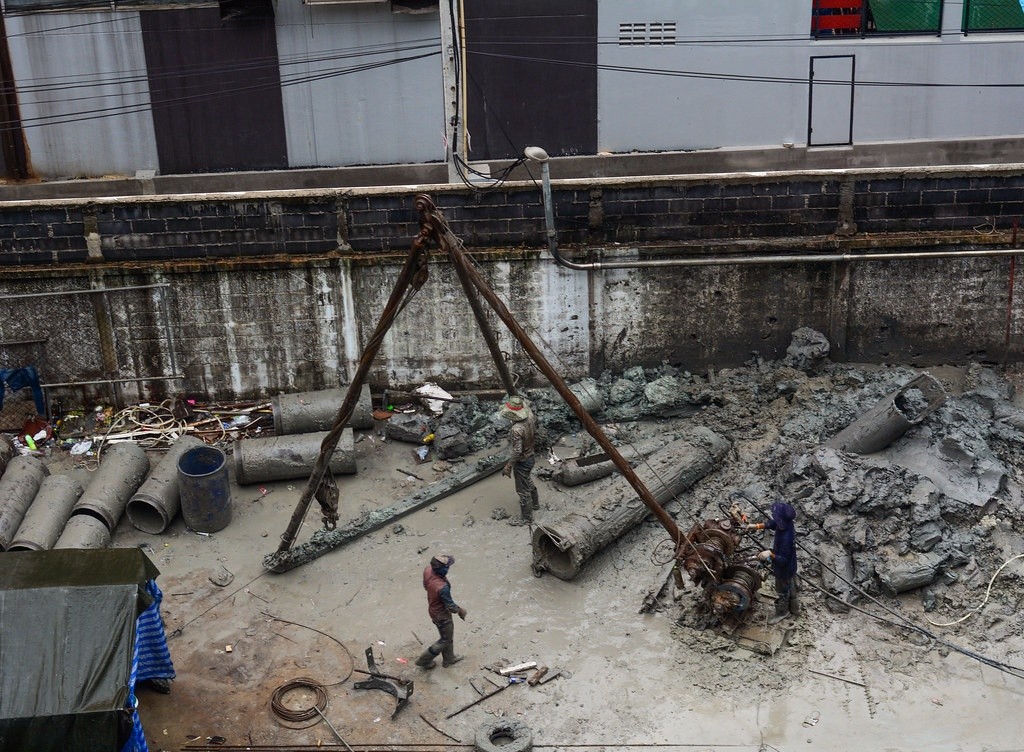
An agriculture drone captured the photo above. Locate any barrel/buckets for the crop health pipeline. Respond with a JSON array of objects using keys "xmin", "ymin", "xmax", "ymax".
[{"xmin": 177, "ymin": 445, "xmax": 232, "ymax": 534}]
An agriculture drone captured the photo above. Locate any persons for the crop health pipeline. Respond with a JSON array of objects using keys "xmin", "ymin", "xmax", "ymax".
[
  {"xmin": 747, "ymin": 503, "xmax": 801, "ymax": 617},
  {"xmin": 415, "ymin": 555, "xmax": 467, "ymax": 669},
  {"xmin": 499, "ymin": 396, "xmax": 540, "ymax": 526}
]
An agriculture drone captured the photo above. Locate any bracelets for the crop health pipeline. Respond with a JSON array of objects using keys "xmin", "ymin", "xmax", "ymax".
[{"xmin": 757, "ymin": 523, "xmax": 759, "ymax": 529}]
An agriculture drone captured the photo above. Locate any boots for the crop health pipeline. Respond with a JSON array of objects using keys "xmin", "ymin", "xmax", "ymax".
[
  {"xmin": 767, "ymin": 598, "xmax": 791, "ymax": 625},
  {"xmin": 442, "ymin": 640, "xmax": 464, "ymax": 667},
  {"xmin": 414, "ymin": 647, "xmax": 438, "ymax": 668},
  {"xmin": 507, "ymin": 492, "xmax": 534, "ymax": 526},
  {"xmin": 530, "ymin": 487, "xmax": 541, "ymax": 509},
  {"xmin": 790, "ymin": 596, "xmax": 802, "ymax": 614}
]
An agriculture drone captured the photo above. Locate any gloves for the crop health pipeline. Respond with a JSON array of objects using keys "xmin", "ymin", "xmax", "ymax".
[
  {"xmin": 758, "ymin": 550, "xmax": 771, "ymax": 562},
  {"xmin": 746, "ymin": 523, "xmax": 759, "ymax": 531}
]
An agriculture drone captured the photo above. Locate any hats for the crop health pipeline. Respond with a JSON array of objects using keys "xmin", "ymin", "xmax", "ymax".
[
  {"xmin": 501, "ymin": 396, "xmax": 528, "ymax": 422},
  {"xmin": 772, "ymin": 502, "xmax": 796, "ymax": 530},
  {"xmin": 431, "ymin": 555, "xmax": 455, "ymax": 567}
]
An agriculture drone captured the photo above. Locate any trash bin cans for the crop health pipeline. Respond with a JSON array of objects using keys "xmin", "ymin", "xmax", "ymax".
[{"xmin": 177, "ymin": 445, "xmax": 232, "ymax": 533}]
